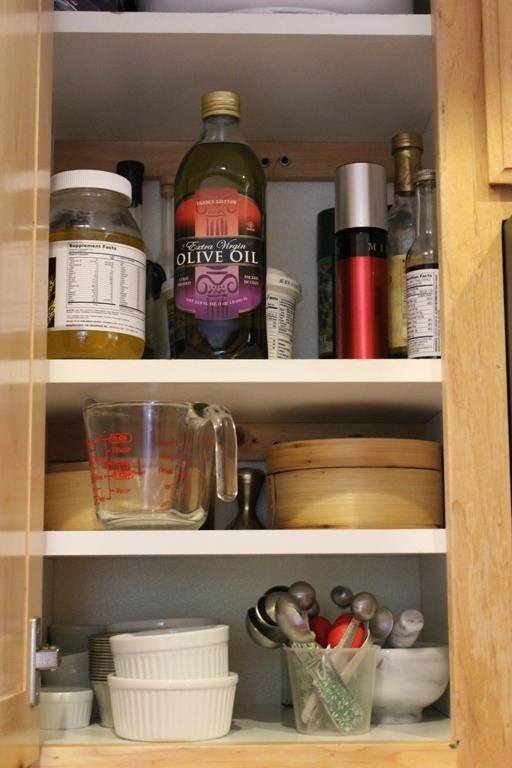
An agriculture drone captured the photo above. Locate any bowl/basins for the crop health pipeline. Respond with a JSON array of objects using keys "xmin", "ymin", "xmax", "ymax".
[
  {"xmin": 372, "ymin": 639, "xmax": 450, "ymax": 724},
  {"xmin": 38, "ymin": 605, "xmax": 239, "ymax": 739}
]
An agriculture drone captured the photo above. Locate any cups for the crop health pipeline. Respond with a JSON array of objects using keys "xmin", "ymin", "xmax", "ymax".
[{"xmin": 83, "ymin": 392, "xmax": 239, "ymax": 525}]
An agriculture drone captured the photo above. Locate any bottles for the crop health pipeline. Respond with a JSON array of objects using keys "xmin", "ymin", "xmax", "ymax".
[
  {"xmin": 171, "ymin": 91, "xmax": 271, "ymax": 362},
  {"xmin": 387, "ymin": 130, "xmax": 441, "ymax": 359}
]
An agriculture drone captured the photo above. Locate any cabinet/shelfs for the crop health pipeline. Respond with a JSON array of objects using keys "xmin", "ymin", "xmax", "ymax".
[{"xmin": 0, "ymin": 0, "xmax": 511, "ymax": 766}]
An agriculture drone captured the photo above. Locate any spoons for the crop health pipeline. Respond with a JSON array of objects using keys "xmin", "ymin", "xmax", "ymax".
[{"xmin": 239, "ymin": 582, "xmax": 400, "ymax": 734}]
